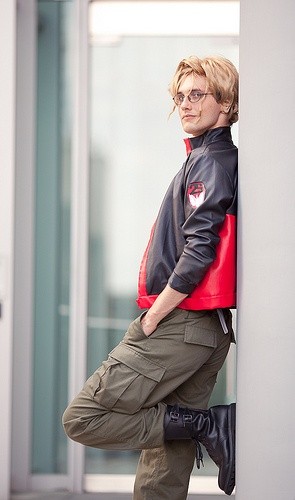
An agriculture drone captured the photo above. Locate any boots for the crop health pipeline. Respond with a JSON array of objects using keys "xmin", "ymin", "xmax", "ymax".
[{"xmin": 165, "ymin": 402, "xmax": 237, "ymax": 495}]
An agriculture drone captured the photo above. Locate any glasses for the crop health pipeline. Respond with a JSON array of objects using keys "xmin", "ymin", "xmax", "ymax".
[{"xmin": 173, "ymin": 91, "xmax": 212, "ymax": 104}]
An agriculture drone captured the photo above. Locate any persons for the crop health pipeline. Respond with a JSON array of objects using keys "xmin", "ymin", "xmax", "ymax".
[{"xmin": 61, "ymin": 56, "xmax": 238, "ymax": 500}]
